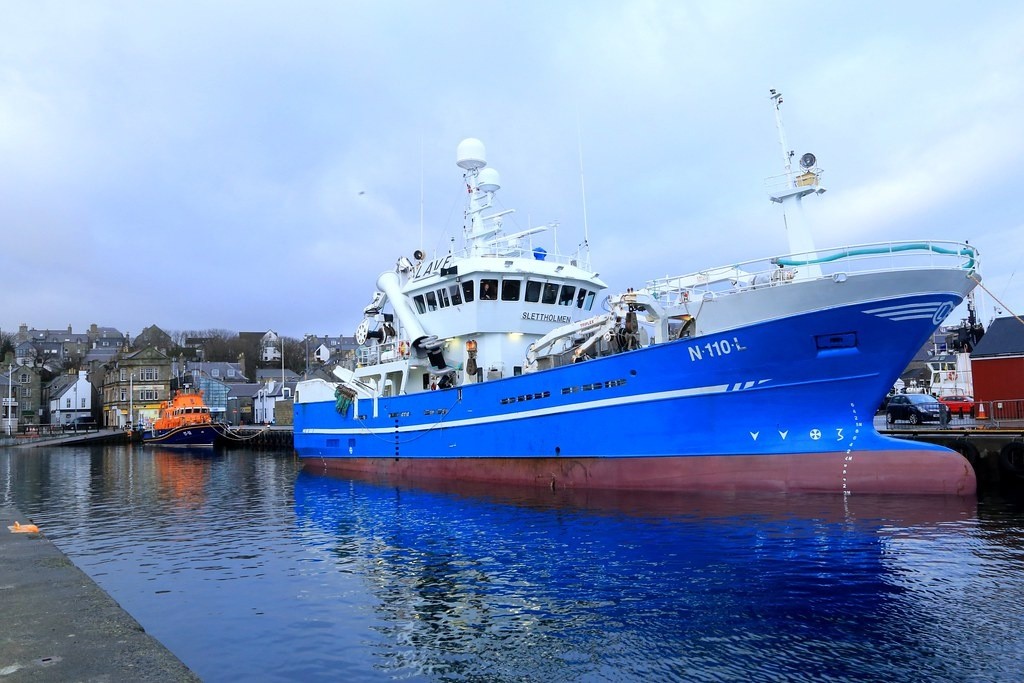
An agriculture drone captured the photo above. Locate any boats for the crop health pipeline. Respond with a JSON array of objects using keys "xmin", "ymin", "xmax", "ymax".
[
  {"xmin": 142, "ymin": 389, "xmax": 226, "ymax": 449},
  {"xmin": 296, "ymin": 92, "xmax": 982, "ymax": 492}
]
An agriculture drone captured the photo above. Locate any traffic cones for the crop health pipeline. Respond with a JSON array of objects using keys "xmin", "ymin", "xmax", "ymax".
[{"xmin": 976, "ymin": 399, "xmax": 989, "ymax": 419}]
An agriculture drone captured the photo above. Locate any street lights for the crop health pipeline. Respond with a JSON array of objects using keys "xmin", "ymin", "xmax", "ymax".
[
  {"xmin": 130, "ymin": 373, "xmax": 135, "ymax": 429},
  {"xmin": 8, "ymin": 363, "xmax": 11, "ymax": 435},
  {"xmin": 304, "ymin": 334, "xmax": 313, "ymax": 370}
]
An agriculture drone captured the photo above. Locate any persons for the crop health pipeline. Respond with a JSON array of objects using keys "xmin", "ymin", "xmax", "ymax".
[
  {"xmin": 615, "ymin": 320, "xmax": 636, "ymax": 352},
  {"xmin": 480, "ymin": 283, "xmax": 491, "ymax": 300}
]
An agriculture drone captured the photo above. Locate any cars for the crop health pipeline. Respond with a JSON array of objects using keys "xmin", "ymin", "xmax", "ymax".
[
  {"xmin": 875, "ymin": 397, "xmax": 892, "ymax": 416},
  {"xmin": 938, "ymin": 395, "xmax": 974, "ymax": 413},
  {"xmin": 900, "ymin": 385, "xmax": 929, "ymax": 394},
  {"xmin": 886, "ymin": 394, "xmax": 952, "ymax": 425},
  {"xmin": 66, "ymin": 417, "xmax": 100, "ymax": 430}
]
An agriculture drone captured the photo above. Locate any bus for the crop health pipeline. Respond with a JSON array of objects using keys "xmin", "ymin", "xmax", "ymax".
[{"xmin": 22, "ymin": 424, "xmax": 55, "ymax": 433}]
